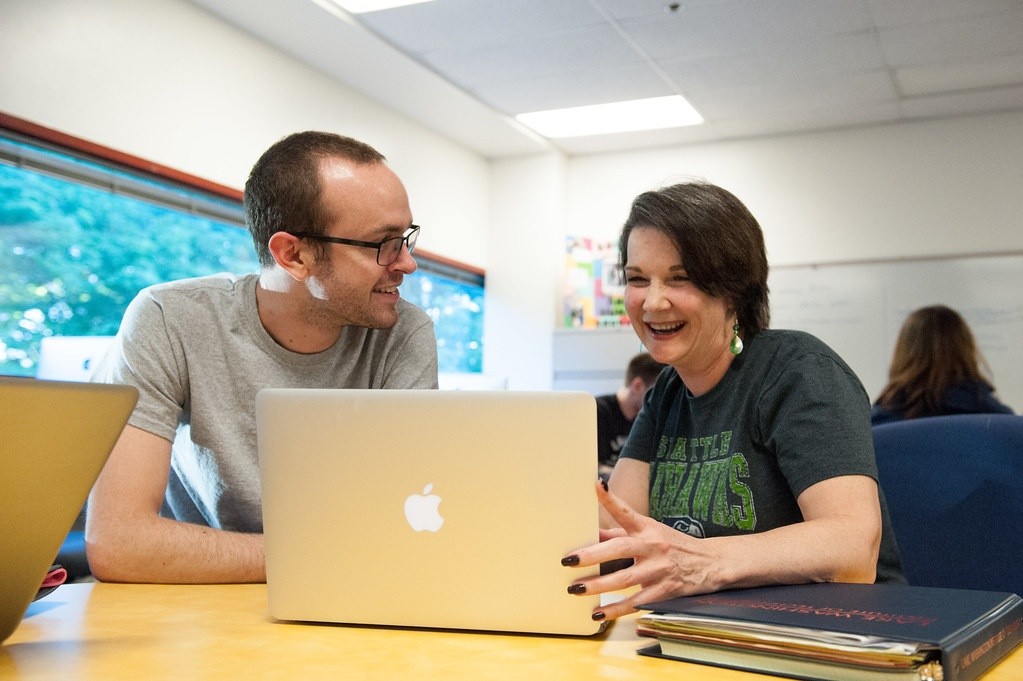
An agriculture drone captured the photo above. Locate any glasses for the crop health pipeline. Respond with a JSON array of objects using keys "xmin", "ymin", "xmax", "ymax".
[{"xmin": 287, "ymin": 225, "xmax": 421, "ymax": 267}]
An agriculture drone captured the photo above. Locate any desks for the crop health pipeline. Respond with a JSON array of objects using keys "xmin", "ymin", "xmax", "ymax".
[{"xmin": 0, "ymin": 566, "xmax": 1023, "ymax": 681}]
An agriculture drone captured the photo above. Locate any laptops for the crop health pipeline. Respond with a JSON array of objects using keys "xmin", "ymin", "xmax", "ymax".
[
  {"xmin": 0, "ymin": 374, "xmax": 140, "ymax": 642},
  {"xmin": 255, "ymin": 387, "xmax": 624, "ymax": 636}
]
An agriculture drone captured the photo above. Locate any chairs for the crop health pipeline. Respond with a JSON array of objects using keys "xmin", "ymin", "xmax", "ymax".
[{"xmin": 872, "ymin": 413, "xmax": 1023, "ymax": 593}]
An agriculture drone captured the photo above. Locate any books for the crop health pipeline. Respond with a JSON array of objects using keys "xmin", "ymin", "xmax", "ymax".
[{"xmin": 633, "ymin": 583, "xmax": 1023, "ymax": 681}]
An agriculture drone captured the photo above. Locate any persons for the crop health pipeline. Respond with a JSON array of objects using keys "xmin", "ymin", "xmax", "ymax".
[
  {"xmin": 562, "ymin": 182, "xmax": 883, "ymax": 624},
  {"xmin": 84, "ymin": 129, "xmax": 440, "ymax": 586},
  {"xmin": 871, "ymin": 307, "xmax": 1015, "ymax": 427},
  {"xmin": 596, "ymin": 352, "xmax": 671, "ymax": 486}
]
